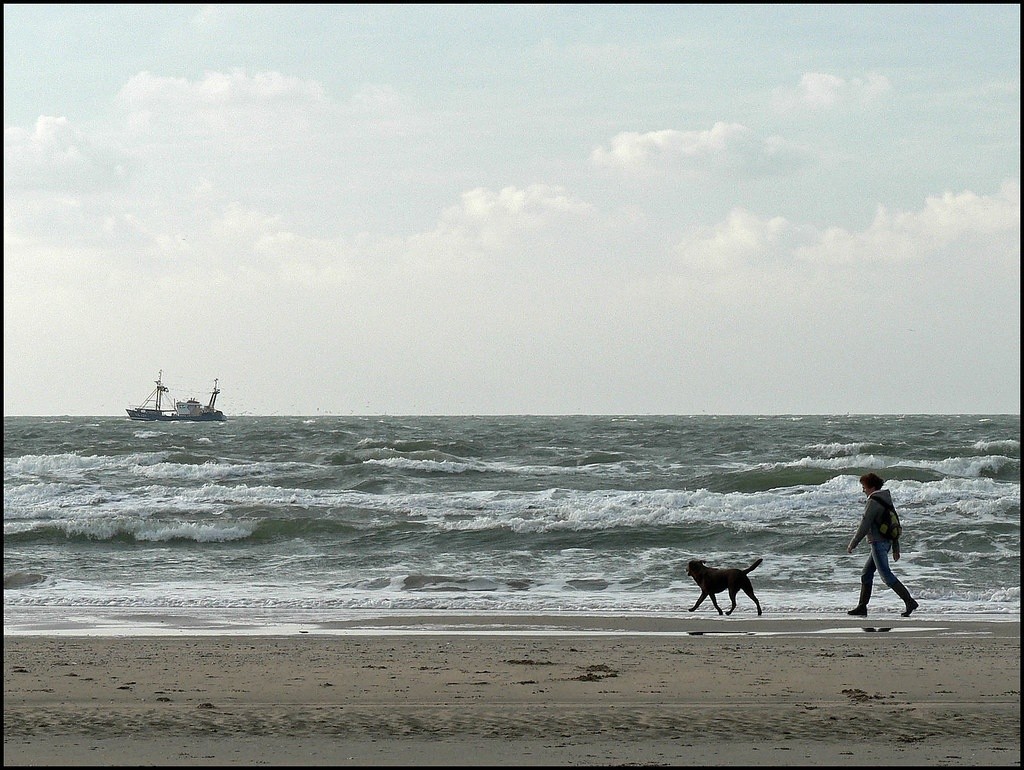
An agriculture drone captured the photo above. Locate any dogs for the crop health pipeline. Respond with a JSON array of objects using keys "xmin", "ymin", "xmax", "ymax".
[{"xmin": 686, "ymin": 558, "xmax": 764, "ymax": 616}]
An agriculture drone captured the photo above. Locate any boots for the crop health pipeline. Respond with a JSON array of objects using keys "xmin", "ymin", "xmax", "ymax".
[
  {"xmin": 847, "ymin": 575, "xmax": 874, "ymax": 615},
  {"xmin": 891, "ymin": 580, "xmax": 919, "ymax": 617}
]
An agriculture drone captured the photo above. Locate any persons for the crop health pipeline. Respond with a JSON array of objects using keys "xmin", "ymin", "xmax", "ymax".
[{"xmin": 847, "ymin": 473, "xmax": 920, "ymax": 618}]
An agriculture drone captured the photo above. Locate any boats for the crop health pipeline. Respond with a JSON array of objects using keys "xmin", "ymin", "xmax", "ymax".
[{"xmin": 125, "ymin": 369, "xmax": 228, "ymax": 422}]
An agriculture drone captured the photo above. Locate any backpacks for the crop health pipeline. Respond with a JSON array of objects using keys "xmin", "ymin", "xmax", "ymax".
[{"xmin": 867, "ymin": 495, "xmax": 902, "ymax": 542}]
